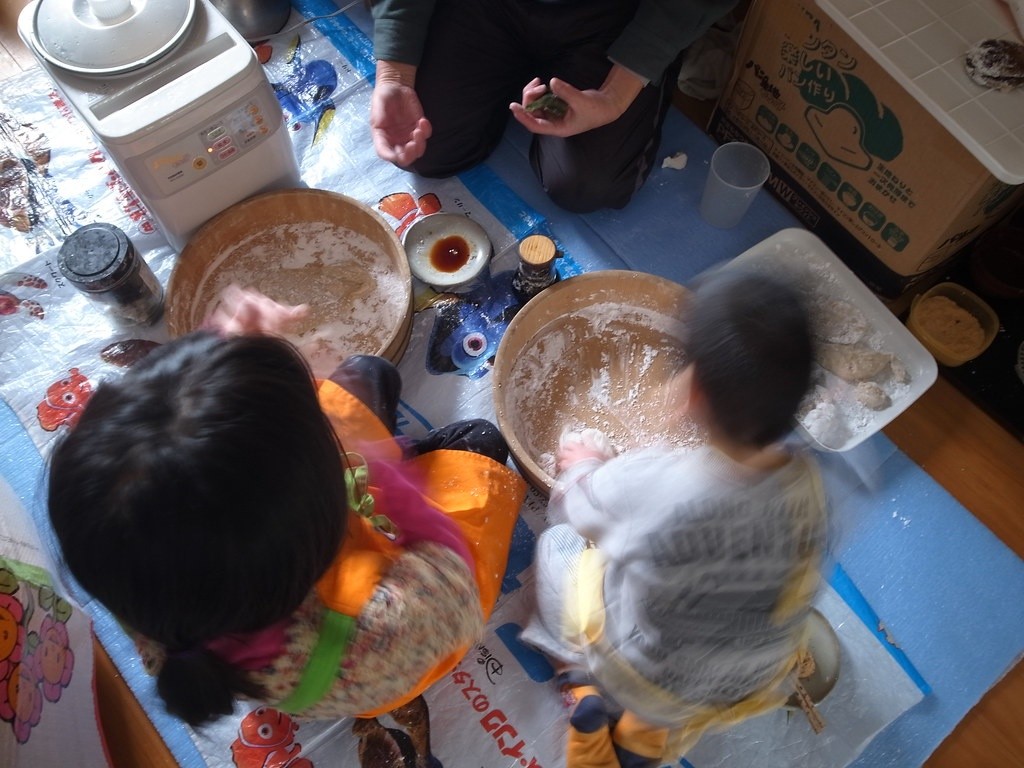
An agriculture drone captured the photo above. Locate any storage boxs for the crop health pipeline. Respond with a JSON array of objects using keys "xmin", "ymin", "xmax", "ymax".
[{"xmin": 722, "ymin": 1, "xmax": 1023, "ymax": 278}]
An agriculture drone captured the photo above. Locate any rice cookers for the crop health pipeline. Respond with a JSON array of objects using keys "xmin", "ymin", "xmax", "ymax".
[{"xmin": 16, "ymin": 0, "xmax": 302, "ymax": 257}]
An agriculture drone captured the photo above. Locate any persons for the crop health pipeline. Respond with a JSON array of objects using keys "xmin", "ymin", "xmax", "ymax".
[
  {"xmin": 514, "ymin": 264, "xmax": 836, "ymax": 768},
  {"xmin": 30, "ymin": 280, "xmax": 530, "ymax": 733},
  {"xmin": 364, "ymin": 0, "xmax": 742, "ymax": 216}
]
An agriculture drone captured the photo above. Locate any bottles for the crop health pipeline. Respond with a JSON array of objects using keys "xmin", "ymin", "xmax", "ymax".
[
  {"xmin": 518, "ymin": 235, "xmax": 557, "ymax": 295},
  {"xmin": 58, "ymin": 223, "xmax": 164, "ymax": 330}
]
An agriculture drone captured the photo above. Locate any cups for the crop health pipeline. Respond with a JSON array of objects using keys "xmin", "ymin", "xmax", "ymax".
[{"xmin": 699, "ymin": 143, "xmax": 771, "ymax": 228}]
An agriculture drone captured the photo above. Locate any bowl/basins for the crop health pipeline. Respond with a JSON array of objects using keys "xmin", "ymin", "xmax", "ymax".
[
  {"xmin": 493, "ymin": 270, "xmax": 711, "ymax": 498},
  {"xmin": 906, "ymin": 282, "xmax": 999, "ymax": 367},
  {"xmin": 164, "ymin": 188, "xmax": 414, "ymax": 378}
]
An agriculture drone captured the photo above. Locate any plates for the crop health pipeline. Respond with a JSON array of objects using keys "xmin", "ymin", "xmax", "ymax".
[{"xmin": 402, "ymin": 212, "xmax": 492, "ymax": 286}]
